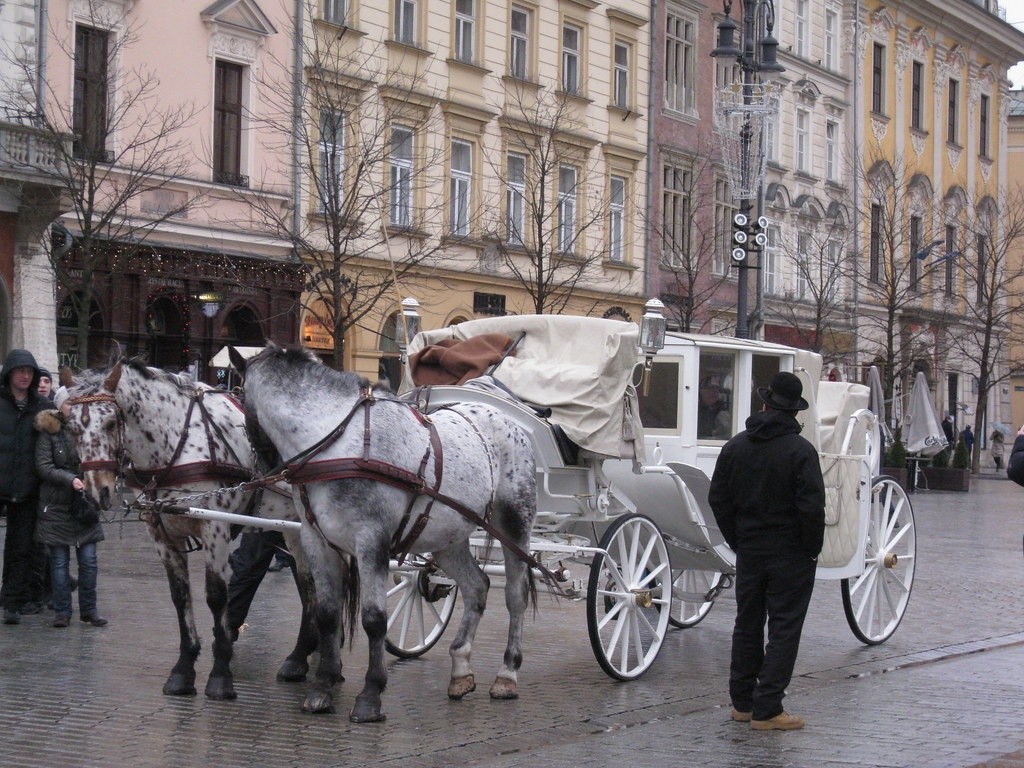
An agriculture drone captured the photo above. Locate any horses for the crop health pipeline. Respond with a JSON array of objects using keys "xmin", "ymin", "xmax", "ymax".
[{"xmin": 52, "ymin": 334, "xmax": 538, "ymax": 725}]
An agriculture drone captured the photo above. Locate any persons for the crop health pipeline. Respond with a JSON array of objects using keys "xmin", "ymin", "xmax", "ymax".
[
  {"xmin": 0, "ymin": 349, "xmax": 78, "ymax": 624},
  {"xmin": 959, "ymin": 425, "xmax": 975, "ymax": 470},
  {"xmin": 990, "ymin": 430, "xmax": 1005, "ymax": 472},
  {"xmin": 1006, "ymin": 423, "xmax": 1024, "ymax": 488},
  {"xmin": 707, "ymin": 371, "xmax": 825, "ymax": 734},
  {"xmin": 941, "ymin": 416, "xmax": 954, "ymax": 466},
  {"xmin": 32, "ymin": 385, "xmax": 106, "ymax": 626},
  {"xmin": 699, "ymin": 376, "xmax": 724, "ymax": 439},
  {"xmin": 878, "ymin": 424, "xmax": 900, "ymax": 529}
]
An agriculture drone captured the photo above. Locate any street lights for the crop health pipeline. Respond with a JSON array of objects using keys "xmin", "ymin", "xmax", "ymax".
[{"xmin": 708, "ymin": 0, "xmax": 786, "ymax": 339}]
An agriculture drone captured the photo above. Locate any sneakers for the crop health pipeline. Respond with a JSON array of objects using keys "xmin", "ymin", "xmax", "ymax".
[{"xmin": 730, "ymin": 709, "xmax": 806, "ymax": 730}]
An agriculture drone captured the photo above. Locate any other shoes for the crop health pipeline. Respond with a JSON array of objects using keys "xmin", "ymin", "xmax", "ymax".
[
  {"xmin": 47, "ymin": 575, "xmax": 78, "ymax": 610},
  {"xmin": 80, "ymin": 615, "xmax": 108, "ymax": 627},
  {"xmin": 53, "ymin": 616, "xmax": 70, "ymax": 628},
  {"xmin": 19, "ymin": 600, "xmax": 44, "ymax": 615},
  {"xmin": 4, "ymin": 610, "xmax": 20, "ymax": 624}
]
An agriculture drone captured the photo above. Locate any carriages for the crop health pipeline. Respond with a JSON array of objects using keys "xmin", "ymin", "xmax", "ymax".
[{"xmin": 57, "ymin": 313, "xmax": 918, "ymax": 724}]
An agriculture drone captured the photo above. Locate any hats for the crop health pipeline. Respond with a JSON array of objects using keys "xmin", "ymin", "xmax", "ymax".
[
  {"xmin": 38, "ymin": 366, "xmax": 52, "ymax": 386},
  {"xmin": 53, "ymin": 385, "xmax": 70, "ymax": 410},
  {"xmin": 757, "ymin": 372, "xmax": 809, "ymax": 411}
]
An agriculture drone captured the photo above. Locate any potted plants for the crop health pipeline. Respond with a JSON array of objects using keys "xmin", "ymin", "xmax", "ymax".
[
  {"xmin": 884, "ymin": 426, "xmax": 908, "ymax": 488},
  {"xmin": 917, "ymin": 433, "xmax": 970, "ymax": 491}
]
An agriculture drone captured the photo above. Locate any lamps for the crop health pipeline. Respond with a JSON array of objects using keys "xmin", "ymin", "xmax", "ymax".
[
  {"xmin": 396, "ymin": 296, "xmax": 421, "ymax": 375},
  {"xmin": 639, "ymin": 297, "xmax": 665, "ymax": 395}
]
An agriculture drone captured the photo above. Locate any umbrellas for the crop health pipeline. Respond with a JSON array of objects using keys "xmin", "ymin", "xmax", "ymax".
[
  {"xmin": 989, "ymin": 421, "xmax": 1011, "ymax": 435},
  {"xmin": 864, "ymin": 365, "xmax": 895, "ymax": 449},
  {"xmin": 899, "ymin": 371, "xmax": 950, "ymax": 491}
]
